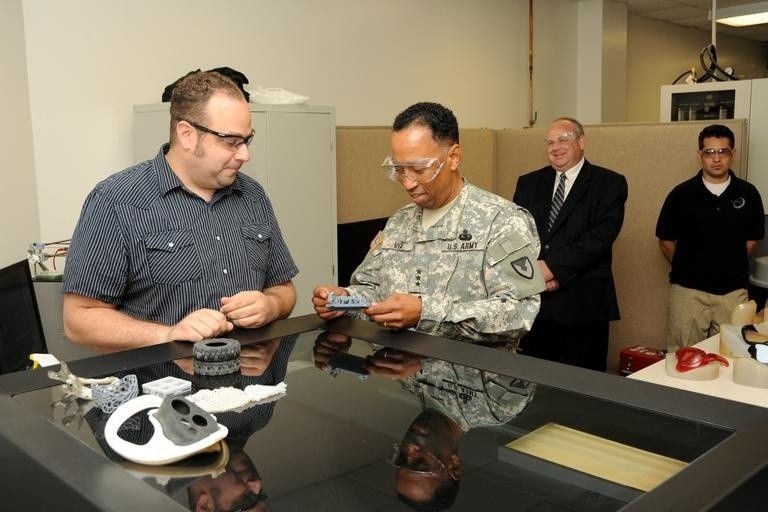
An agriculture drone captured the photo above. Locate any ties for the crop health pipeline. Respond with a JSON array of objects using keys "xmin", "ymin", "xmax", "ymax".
[{"xmin": 547, "ymin": 173, "xmax": 566, "ymax": 234}]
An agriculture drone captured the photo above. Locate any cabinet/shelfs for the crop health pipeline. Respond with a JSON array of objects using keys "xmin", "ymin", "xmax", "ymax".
[
  {"xmin": 659, "ymin": 80, "xmax": 753, "ymax": 184},
  {"xmin": 129, "ymin": 102, "xmax": 338, "ymax": 314},
  {"xmin": 747, "ymin": 78, "xmax": 768, "ymax": 216}
]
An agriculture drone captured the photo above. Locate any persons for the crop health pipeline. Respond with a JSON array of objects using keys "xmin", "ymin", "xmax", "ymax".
[
  {"xmin": 310, "ymin": 102, "xmax": 543, "ymax": 353},
  {"xmin": 74, "ymin": 332, "xmax": 304, "ymax": 512},
  {"xmin": 312, "ymin": 329, "xmax": 537, "ymax": 512},
  {"xmin": 510, "ymin": 118, "xmax": 629, "ymax": 374},
  {"xmin": 59, "ymin": 71, "xmax": 297, "ymax": 357},
  {"xmin": 652, "ymin": 124, "xmax": 766, "ymax": 356}
]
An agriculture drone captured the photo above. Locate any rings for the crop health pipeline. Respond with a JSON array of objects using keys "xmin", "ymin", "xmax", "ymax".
[
  {"xmin": 383, "ymin": 352, "xmax": 388, "ymax": 359},
  {"xmin": 383, "ymin": 321, "xmax": 387, "ymax": 328}
]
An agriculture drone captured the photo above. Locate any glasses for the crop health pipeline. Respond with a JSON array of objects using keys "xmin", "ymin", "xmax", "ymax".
[
  {"xmin": 230, "ymin": 488, "xmax": 268, "ymax": 512},
  {"xmin": 381, "ymin": 147, "xmax": 450, "ymax": 184},
  {"xmin": 187, "ymin": 121, "xmax": 255, "ymax": 148},
  {"xmin": 385, "ymin": 442, "xmax": 452, "ymax": 480},
  {"xmin": 702, "ymin": 148, "xmax": 731, "ymax": 159},
  {"xmin": 543, "ymin": 131, "xmax": 582, "ymax": 147}
]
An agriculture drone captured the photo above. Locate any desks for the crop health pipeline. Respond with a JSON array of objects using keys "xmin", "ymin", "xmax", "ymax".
[
  {"xmin": 0, "ymin": 306, "xmax": 768, "ymax": 511},
  {"xmin": 622, "ymin": 316, "xmax": 768, "ymax": 410}
]
